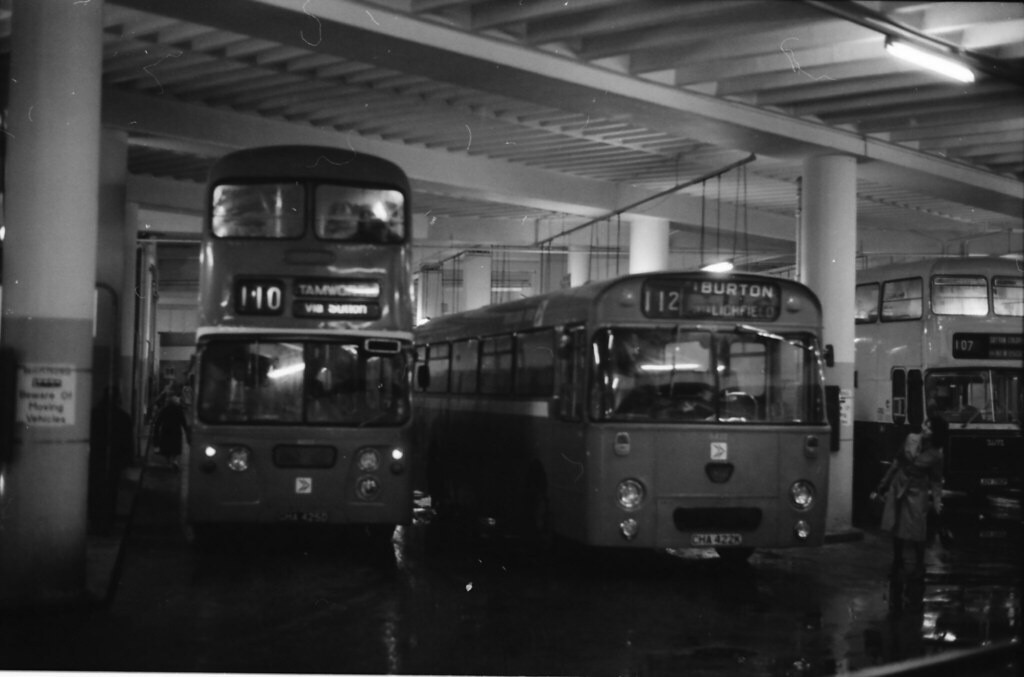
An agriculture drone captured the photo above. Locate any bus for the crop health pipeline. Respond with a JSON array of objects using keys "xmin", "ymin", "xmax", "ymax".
[
  {"xmin": 412, "ymin": 268, "xmax": 838, "ymax": 567},
  {"xmin": 659, "ymin": 253, "xmax": 1024, "ymax": 468},
  {"xmin": 173, "ymin": 142, "xmax": 432, "ymax": 555}
]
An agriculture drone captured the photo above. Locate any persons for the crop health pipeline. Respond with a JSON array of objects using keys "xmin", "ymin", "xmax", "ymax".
[
  {"xmin": 153, "ymin": 367, "xmax": 191, "ymax": 481},
  {"xmin": 867, "ymin": 414, "xmax": 953, "ymax": 630},
  {"xmin": 90, "ymin": 387, "xmax": 136, "ymax": 519},
  {"xmin": 347, "ymin": 211, "xmax": 398, "ymax": 242}
]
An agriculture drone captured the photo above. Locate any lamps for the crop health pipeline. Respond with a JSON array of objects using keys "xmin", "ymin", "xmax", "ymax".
[{"xmin": 884, "ymin": 33, "xmax": 983, "ymax": 85}]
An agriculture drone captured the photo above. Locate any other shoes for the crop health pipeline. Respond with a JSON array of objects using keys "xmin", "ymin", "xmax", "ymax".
[
  {"xmin": 908, "ymin": 564, "xmax": 926, "ymax": 582},
  {"xmin": 886, "ymin": 558, "xmax": 902, "ymax": 578}
]
